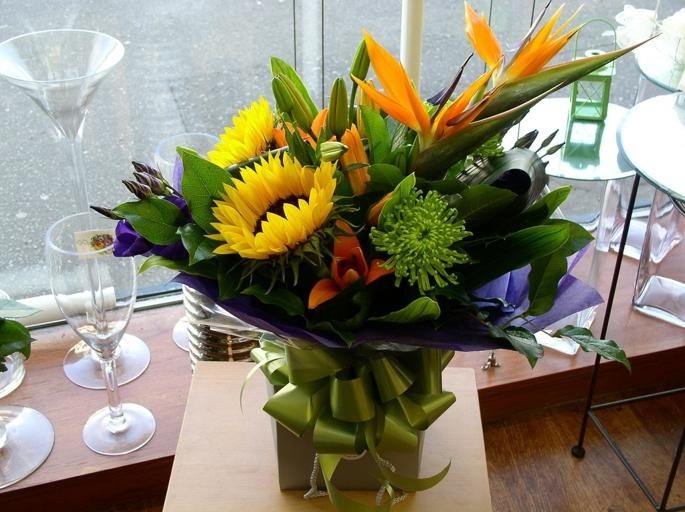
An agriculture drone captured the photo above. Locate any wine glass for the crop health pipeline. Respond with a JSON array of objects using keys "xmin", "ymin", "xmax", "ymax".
[
  {"xmin": 160, "ymin": 131, "xmax": 223, "ymax": 356},
  {"xmin": 0, "ymin": 25, "xmax": 156, "ymax": 392},
  {"xmin": 44, "ymin": 209, "xmax": 158, "ymax": 457}
]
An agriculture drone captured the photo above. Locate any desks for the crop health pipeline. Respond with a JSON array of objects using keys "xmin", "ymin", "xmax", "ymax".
[{"xmin": 572, "ymin": 90, "xmax": 685, "ymax": 512}]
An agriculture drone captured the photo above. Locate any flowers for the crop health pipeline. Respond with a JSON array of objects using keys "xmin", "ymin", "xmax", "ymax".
[{"xmin": 77, "ymin": 1, "xmax": 665, "ymax": 374}]
